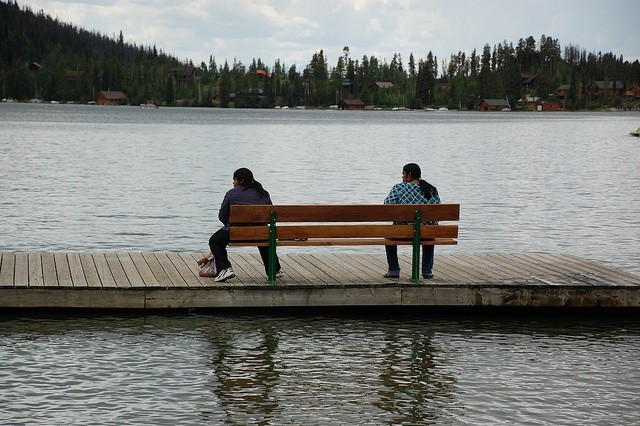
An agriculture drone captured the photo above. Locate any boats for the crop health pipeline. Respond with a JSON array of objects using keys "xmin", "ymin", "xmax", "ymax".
[
  {"xmin": 629, "ymin": 127, "xmax": 640, "ymax": 136},
  {"xmin": 140, "ymin": 100, "xmax": 157, "ymax": 107}
]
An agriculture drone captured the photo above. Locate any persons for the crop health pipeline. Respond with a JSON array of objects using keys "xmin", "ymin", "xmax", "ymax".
[
  {"xmin": 209, "ymin": 167, "xmax": 284, "ymax": 282},
  {"xmin": 383, "ymin": 163, "xmax": 441, "ymax": 279}
]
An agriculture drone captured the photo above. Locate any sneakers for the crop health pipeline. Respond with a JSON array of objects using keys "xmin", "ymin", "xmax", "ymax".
[
  {"xmin": 422, "ymin": 272, "xmax": 433, "ymax": 279},
  {"xmin": 214, "ymin": 267, "xmax": 235, "ymax": 282},
  {"xmin": 383, "ymin": 270, "xmax": 399, "ymax": 278},
  {"xmin": 275, "ymin": 269, "xmax": 284, "ymax": 278}
]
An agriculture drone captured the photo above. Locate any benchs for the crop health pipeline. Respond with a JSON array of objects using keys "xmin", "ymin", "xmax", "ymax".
[{"xmin": 226, "ymin": 204, "xmax": 460, "ymax": 283}]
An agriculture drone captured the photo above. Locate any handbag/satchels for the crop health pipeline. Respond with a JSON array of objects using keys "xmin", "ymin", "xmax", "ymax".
[{"xmin": 197, "ymin": 252, "xmax": 218, "ymax": 278}]
